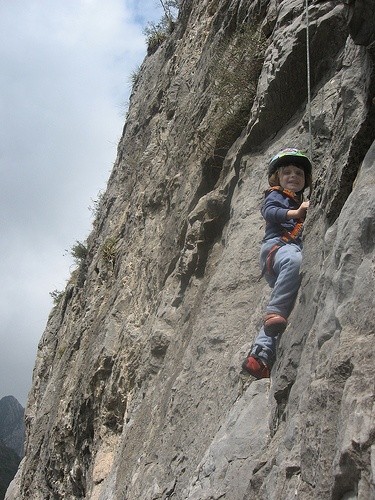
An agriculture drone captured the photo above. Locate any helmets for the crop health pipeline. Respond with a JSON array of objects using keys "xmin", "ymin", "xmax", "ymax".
[{"xmin": 267, "ymin": 148, "xmax": 311, "ymax": 179}]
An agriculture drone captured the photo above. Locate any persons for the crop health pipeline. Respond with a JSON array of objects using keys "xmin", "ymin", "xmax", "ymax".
[{"xmin": 242, "ymin": 148, "xmax": 313, "ymax": 379}]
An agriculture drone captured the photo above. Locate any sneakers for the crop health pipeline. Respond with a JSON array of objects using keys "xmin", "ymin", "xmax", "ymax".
[
  {"xmin": 263, "ymin": 313, "xmax": 287, "ymax": 337},
  {"xmin": 242, "ymin": 356, "xmax": 269, "ymax": 379}
]
